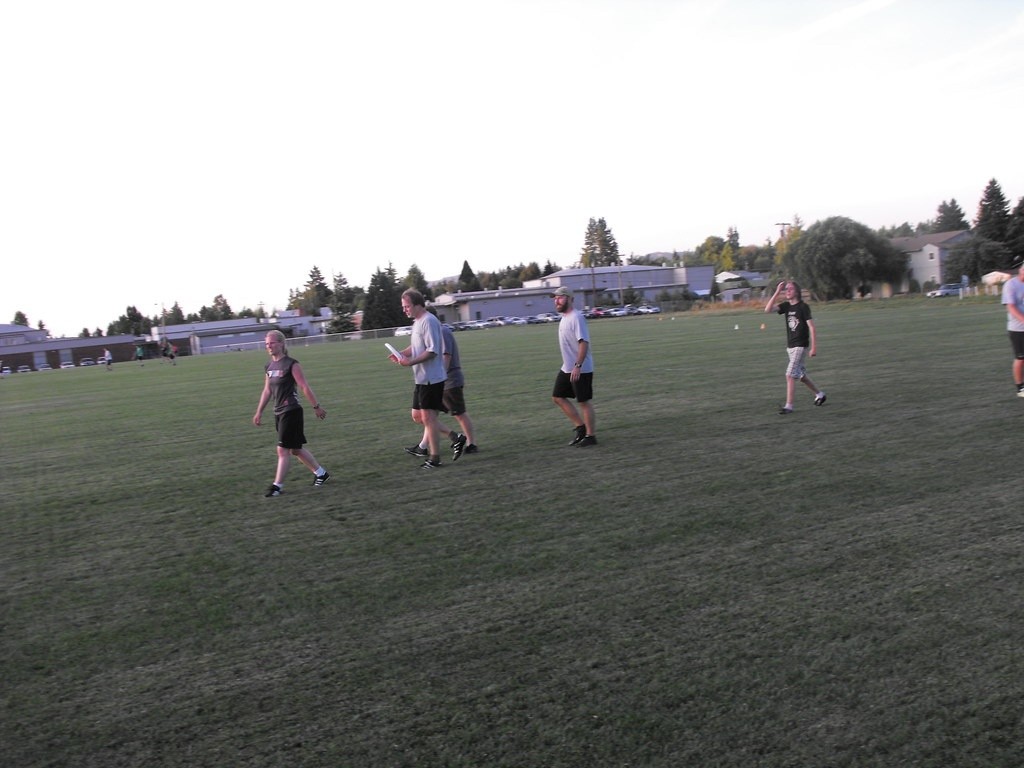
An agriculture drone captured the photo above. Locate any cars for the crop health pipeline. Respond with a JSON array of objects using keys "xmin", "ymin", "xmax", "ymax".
[
  {"xmin": 394, "ymin": 304, "xmax": 661, "ymax": 337},
  {"xmin": 1, "ymin": 355, "xmax": 106, "ymax": 374},
  {"xmin": 926, "ymin": 283, "xmax": 963, "ymax": 298}
]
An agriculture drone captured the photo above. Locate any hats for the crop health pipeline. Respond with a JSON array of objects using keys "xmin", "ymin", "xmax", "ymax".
[
  {"xmin": 1010, "ymin": 255, "xmax": 1024, "ymax": 270},
  {"xmin": 549, "ymin": 286, "xmax": 575, "ymax": 298}
]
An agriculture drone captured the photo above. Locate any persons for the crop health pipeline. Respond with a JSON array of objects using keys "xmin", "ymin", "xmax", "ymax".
[
  {"xmin": 104, "ymin": 347, "xmax": 113, "ymax": 371},
  {"xmin": 135, "ymin": 345, "xmax": 144, "ymax": 367},
  {"xmin": 253, "ymin": 330, "xmax": 331, "ymax": 498},
  {"xmin": 160, "ymin": 341, "xmax": 177, "ymax": 366},
  {"xmin": 403, "ymin": 305, "xmax": 478, "ymax": 456},
  {"xmin": 1001, "ymin": 262, "xmax": 1024, "ymax": 399},
  {"xmin": 548, "ymin": 286, "xmax": 598, "ymax": 447},
  {"xmin": 386, "ymin": 288, "xmax": 467, "ymax": 470},
  {"xmin": 765, "ymin": 281, "xmax": 827, "ymax": 416}
]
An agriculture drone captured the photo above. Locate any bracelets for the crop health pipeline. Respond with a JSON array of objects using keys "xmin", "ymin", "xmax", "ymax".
[
  {"xmin": 313, "ymin": 402, "xmax": 320, "ymax": 409},
  {"xmin": 574, "ymin": 363, "xmax": 581, "ymax": 368}
]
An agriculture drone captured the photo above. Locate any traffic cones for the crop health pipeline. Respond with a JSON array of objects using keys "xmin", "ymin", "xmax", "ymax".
[{"xmin": 760, "ymin": 324, "xmax": 765, "ymax": 331}]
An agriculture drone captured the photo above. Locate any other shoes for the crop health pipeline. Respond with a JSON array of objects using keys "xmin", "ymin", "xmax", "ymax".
[{"xmin": 1017, "ymin": 386, "xmax": 1024, "ymax": 397}]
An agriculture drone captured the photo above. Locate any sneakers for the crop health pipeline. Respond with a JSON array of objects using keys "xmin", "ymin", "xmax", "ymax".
[
  {"xmin": 463, "ymin": 444, "xmax": 479, "ymax": 455},
  {"xmin": 450, "ymin": 432, "xmax": 466, "ymax": 461},
  {"xmin": 778, "ymin": 409, "xmax": 792, "ymax": 416},
  {"xmin": 416, "ymin": 459, "xmax": 443, "ymax": 470},
  {"xmin": 265, "ymin": 485, "xmax": 288, "ymax": 497},
  {"xmin": 312, "ymin": 471, "xmax": 331, "ymax": 488},
  {"xmin": 576, "ymin": 435, "xmax": 597, "ymax": 448},
  {"xmin": 569, "ymin": 424, "xmax": 586, "ymax": 446},
  {"xmin": 404, "ymin": 445, "xmax": 428, "ymax": 456},
  {"xmin": 813, "ymin": 394, "xmax": 826, "ymax": 406}
]
